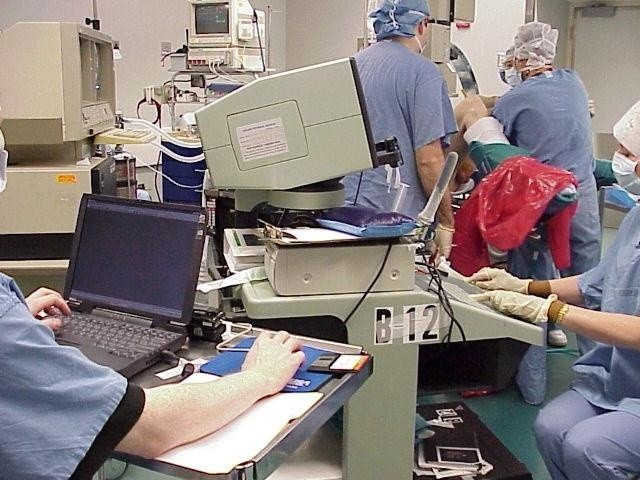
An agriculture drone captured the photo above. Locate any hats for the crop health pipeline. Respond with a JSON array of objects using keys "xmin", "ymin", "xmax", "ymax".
[{"xmin": 612, "ymin": 100, "xmax": 640, "ymax": 159}]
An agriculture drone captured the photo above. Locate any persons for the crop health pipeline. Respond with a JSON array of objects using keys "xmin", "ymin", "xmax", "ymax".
[
  {"xmin": 464, "ymin": 95, "xmax": 639, "ymax": 479},
  {"xmin": 440, "ymin": 22, "xmax": 602, "ymax": 407},
  {"xmin": 0, "ymin": 129, "xmax": 307, "ymax": 480},
  {"xmin": 340, "ymin": 0, "xmax": 461, "ymax": 266}
]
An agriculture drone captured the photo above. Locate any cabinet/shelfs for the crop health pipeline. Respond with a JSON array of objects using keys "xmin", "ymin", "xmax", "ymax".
[{"xmin": 99, "ymin": 325, "xmax": 374, "ymax": 480}]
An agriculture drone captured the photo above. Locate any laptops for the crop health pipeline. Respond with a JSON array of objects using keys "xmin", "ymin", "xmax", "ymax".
[{"xmin": 39, "ymin": 192, "xmax": 209, "ymax": 379}]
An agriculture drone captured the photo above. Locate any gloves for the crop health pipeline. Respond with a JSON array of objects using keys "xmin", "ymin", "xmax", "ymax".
[
  {"xmin": 430, "ymin": 223, "xmax": 456, "ymax": 267},
  {"xmin": 467, "ymin": 289, "xmax": 558, "ymax": 324},
  {"xmin": 464, "ymin": 266, "xmax": 532, "ymax": 295}
]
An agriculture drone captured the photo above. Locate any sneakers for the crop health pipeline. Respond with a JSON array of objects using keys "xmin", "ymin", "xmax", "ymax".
[{"xmin": 548, "ymin": 328, "xmax": 567, "ymax": 346}]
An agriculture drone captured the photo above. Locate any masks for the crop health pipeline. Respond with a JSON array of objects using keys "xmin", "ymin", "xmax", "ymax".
[
  {"xmin": 505, "ymin": 66, "xmax": 529, "ymax": 86},
  {"xmin": 611, "ymin": 151, "xmax": 640, "ymax": 196}
]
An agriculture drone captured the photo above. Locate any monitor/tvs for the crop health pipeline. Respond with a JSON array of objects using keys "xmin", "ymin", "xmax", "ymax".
[{"xmin": 189, "ymin": 0, "xmax": 254, "ymax": 45}]
